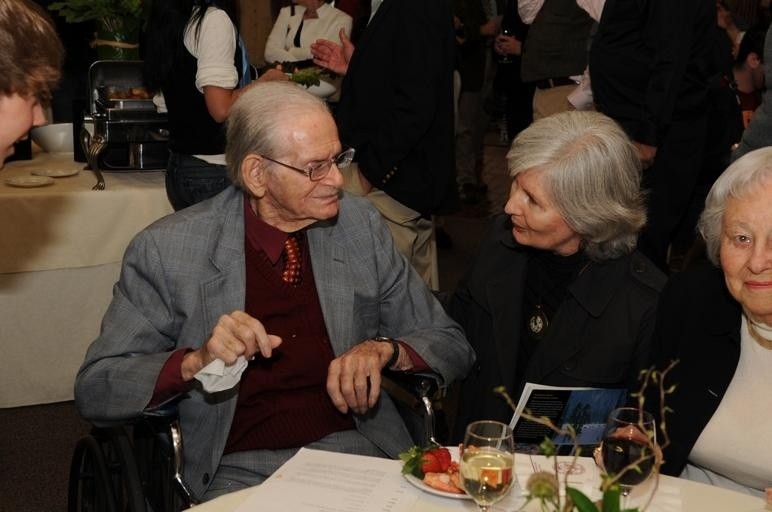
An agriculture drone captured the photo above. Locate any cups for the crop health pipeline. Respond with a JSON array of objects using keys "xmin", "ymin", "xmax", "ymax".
[{"xmin": 461, "ymin": 420, "xmax": 516, "ymax": 511}]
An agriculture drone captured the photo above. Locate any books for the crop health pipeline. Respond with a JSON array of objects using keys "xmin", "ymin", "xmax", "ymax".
[{"xmin": 509, "ymin": 382, "xmax": 627, "ymax": 459}]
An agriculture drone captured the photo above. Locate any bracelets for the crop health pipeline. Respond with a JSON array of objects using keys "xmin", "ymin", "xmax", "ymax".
[{"xmin": 372, "ymin": 336, "xmax": 400, "ymax": 370}]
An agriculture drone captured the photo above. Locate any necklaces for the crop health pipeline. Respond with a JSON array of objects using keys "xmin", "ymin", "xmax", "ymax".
[{"xmin": 527, "ymin": 253, "xmax": 568, "ymax": 336}]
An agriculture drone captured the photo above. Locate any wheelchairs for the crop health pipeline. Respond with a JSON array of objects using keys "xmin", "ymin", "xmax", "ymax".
[{"xmin": 67, "ymin": 345, "xmax": 447, "ymax": 511}]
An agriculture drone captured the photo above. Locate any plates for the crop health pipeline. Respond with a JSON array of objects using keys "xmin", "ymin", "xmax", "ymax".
[
  {"xmin": 32, "ymin": 165, "xmax": 80, "ymax": 178},
  {"xmin": 4, "ymin": 174, "xmax": 53, "ymax": 189},
  {"xmin": 400, "ymin": 445, "xmax": 476, "ymax": 501}
]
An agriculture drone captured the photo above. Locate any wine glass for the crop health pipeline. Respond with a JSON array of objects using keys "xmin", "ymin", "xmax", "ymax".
[
  {"xmin": 601, "ymin": 405, "xmax": 658, "ymax": 511},
  {"xmin": 498, "ymin": 28, "xmax": 512, "ymax": 65}
]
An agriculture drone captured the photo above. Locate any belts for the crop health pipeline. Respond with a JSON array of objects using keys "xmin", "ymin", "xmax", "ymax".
[{"xmin": 537, "ymin": 76, "xmax": 574, "ymax": 90}]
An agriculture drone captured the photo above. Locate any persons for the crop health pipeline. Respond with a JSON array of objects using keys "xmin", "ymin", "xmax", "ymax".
[
  {"xmin": 0, "ymin": 2, "xmax": 65, "ymax": 170},
  {"xmin": 594, "ymin": 144, "xmax": 771, "ymax": 512},
  {"xmin": 445, "ymin": 110, "xmax": 668, "ymax": 456},
  {"xmin": 141, "ymin": 2, "xmax": 771, "ymax": 293},
  {"xmin": 73, "ymin": 80, "xmax": 478, "ymax": 504}
]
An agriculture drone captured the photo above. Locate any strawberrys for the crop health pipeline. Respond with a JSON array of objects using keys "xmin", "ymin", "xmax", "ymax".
[{"xmin": 420, "ymin": 447, "xmax": 451, "ymax": 473}]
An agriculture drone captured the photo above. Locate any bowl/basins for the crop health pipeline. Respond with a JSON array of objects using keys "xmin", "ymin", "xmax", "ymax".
[{"xmin": 282, "ymin": 72, "xmax": 337, "ymax": 99}]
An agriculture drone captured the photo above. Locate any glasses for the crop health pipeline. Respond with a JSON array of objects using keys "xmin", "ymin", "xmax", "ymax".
[{"xmin": 264, "ymin": 148, "xmax": 355, "ymax": 181}]
